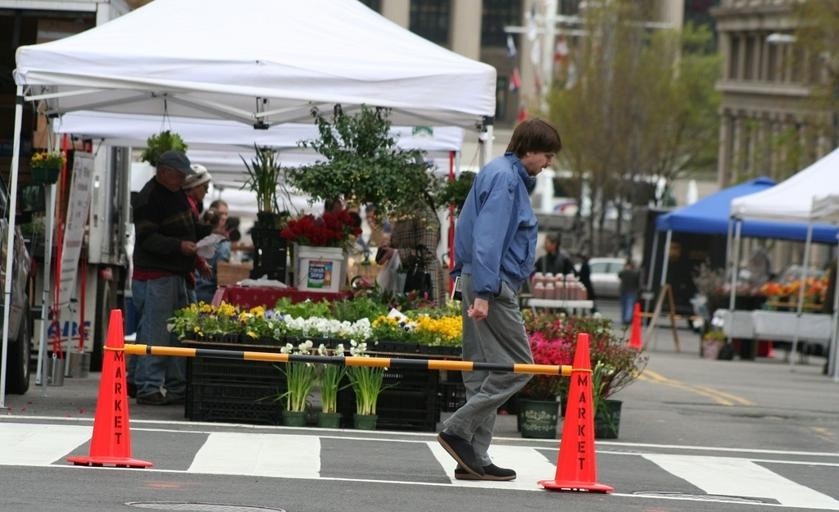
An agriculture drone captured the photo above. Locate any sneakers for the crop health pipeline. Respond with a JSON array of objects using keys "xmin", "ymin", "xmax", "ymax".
[
  {"xmin": 432, "ymin": 428, "xmax": 518, "ymax": 486},
  {"xmin": 127, "ymin": 382, "xmax": 184, "ymax": 407}
]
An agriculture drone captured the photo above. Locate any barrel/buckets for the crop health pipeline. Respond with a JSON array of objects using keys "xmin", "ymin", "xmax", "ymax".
[
  {"xmin": 297, "ymin": 245, "xmax": 344, "ymax": 292},
  {"xmin": 40, "ymin": 358, "xmax": 64, "ymax": 387},
  {"xmin": 515, "ymin": 398, "xmax": 559, "ymax": 439},
  {"xmin": 69, "ymin": 351, "xmax": 90, "ymax": 378}
]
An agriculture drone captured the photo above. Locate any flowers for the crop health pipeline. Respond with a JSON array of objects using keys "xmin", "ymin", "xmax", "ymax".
[
  {"xmin": 29, "ymin": 148, "xmax": 66, "ymax": 171},
  {"xmin": 279, "ymin": 203, "xmax": 363, "ymax": 254},
  {"xmin": 522, "ymin": 308, "xmax": 649, "ymax": 401},
  {"xmin": 166, "ymin": 290, "xmax": 466, "ymax": 365},
  {"xmin": 700, "ymin": 276, "xmax": 831, "ymax": 358}
]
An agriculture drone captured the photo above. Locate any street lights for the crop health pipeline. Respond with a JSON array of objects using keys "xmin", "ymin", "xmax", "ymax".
[{"xmin": 767, "ymin": 32, "xmax": 839, "ymax": 77}]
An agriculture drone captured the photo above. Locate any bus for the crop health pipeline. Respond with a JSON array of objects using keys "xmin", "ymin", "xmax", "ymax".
[{"xmin": 534, "ymin": 164, "xmax": 676, "ymax": 229}]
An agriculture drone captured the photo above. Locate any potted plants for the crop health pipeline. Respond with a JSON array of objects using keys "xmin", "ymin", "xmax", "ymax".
[{"xmin": 230, "ymin": 140, "xmax": 300, "ymax": 224}]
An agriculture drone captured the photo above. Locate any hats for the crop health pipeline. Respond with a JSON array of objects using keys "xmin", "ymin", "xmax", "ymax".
[
  {"xmin": 182, "ymin": 161, "xmax": 215, "ymax": 189},
  {"xmin": 157, "ymin": 150, "xmax": 196, "ymax": 177}
]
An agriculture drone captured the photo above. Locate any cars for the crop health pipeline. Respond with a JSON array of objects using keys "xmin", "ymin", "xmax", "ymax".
[
  {"xmin": 570, "ymin": 255, "xmax": 633, "ymax": 299},
  {"xmin": 1, "ymin": 175, "xmax": 46, "ymax": 396}
]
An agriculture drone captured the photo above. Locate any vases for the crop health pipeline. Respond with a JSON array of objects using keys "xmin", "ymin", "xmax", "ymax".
[
  {"xmin": 517, "ymin": 396, "xmax": 567, "ymax": 439},
  {"xmin": 282, "ymin": 409, "xmax": 307, "ymax": 426},
  {"xmin": 27, "ymin": 166, "xmax": 61, "ymax": 186},
  {"xmin": 353, "ymin": 413, "xmax": 379, "ymax": 430},
  {"xmin": 317, "ymin": 411, "xmax": 342, "ymax": 428}
]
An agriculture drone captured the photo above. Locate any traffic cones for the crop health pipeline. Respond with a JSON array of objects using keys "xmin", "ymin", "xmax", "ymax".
[
  {"xmin": 64, "ymin": 306, "xmax": 159, "ymax": 467},
  {"xmin": 629, "ymin": 301, "xmax": 645, "ymax": 351},
  {"xmin": 534, "ymin": 329, "xmax": 618, "ymax": 493}
]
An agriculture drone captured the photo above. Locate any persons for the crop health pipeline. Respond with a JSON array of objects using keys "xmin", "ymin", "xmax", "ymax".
[
  {"xmin": 534, "ymin": 234, "xmax": 573, "ymax": 275},
  {"xmin": 619, "ymin": 262, "xmax": 639, "ymax": 326},
  {"xmin": 324, "ymin": 193, "xmax": 446, "ymax": 306},
  {"xmin": 573, "ymin": 255, "xmax": 601, "ymax": 320},
  {"xmin": 132, "ymin": 152, "xmax": 243, "ymax": 406},
  {"xmin": 438, "ymin": 119, "xmax": 561, "ymax": 480}
]
